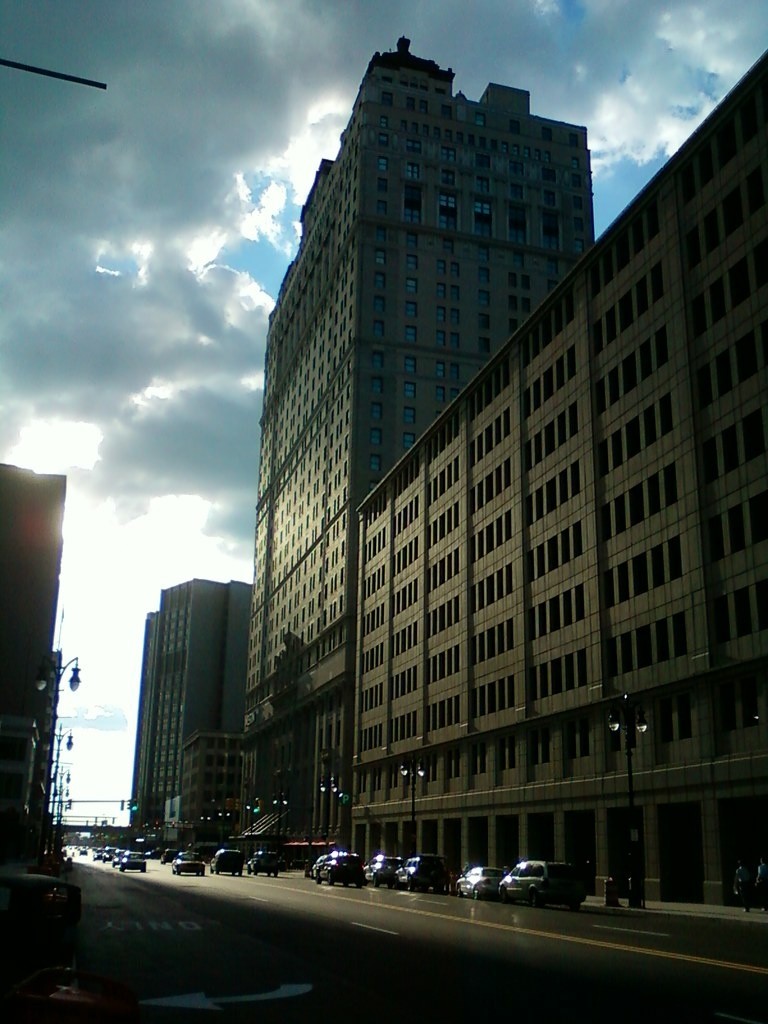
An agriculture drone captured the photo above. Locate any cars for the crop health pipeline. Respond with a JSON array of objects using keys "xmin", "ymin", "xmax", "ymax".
[
  {"xmin": 171, "ymin": 851, "xmax": 205, "ymax": 877},
  {"xmin": 455, "ymin": 867, "xmax": 505, "ymax": 900},
  {"xmin": 66, "ymin": 844, "xmax": 178, "ymax": 873}
]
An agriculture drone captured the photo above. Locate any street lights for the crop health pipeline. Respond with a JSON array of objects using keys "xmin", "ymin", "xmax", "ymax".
[
  {"xmin": 272, "ymin": 786, "xmax": 290, "ymax": 851},
  {"xmin": 401, "ymin": 754, "xmax": 426, "ymax": 861},
  {"xmin": 609, "ymin": 692, "xmax": 650, "ymax": 909},
  {"xmin": 91, "ymin": 793, "xmax": 262, "ymax": 852},
  {"xmin": 319, "ymin": 770, "xmax": 343, "ymax": 852},
  {"xmin": 31, "ymin": 645, "xmax": 83, "ymax": 877}
]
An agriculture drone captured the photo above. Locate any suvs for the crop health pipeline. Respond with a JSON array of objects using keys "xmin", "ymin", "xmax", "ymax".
[
  {"xmin": 314, "ymin": 852, "xmax": 363, "ymax": 887},
  {"xmin": 211, "ymin": 849, "xmax": 243, "ymax": 876},
  {"xmin": 389, "ymin": 856, "xmax": 451, "ymax": 892},
  {"xmin": 498, "ymin": 860, "xmax": 587, "ymax": 909},
  {"xmin": 361, "ymin": 855, "xmax": 404, "ymax": 888},
  {"xmin": 246, "ymin": 851, "xmax": 279, "ymax": 877}
]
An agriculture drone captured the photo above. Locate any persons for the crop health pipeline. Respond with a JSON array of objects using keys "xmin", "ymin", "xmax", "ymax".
[
  {"xmin": 63, "ymin": 858, "xmax": 74, "ymax": 883},
  {"xmin": 733, "ymin": 860, "xmax": 751, "ymax": 912},
  {"xmin": 754, "ymin": 857, "xmax": 768, "ymax": 911}
]
